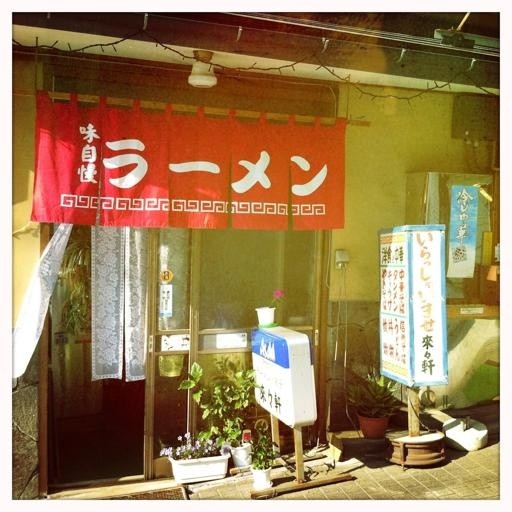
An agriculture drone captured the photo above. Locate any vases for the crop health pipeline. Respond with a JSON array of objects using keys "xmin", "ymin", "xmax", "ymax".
[
  {"xmin": 167, "ymin": 453, "xmax": 231, "ymax": 482},
  {"xmin": 356, "ymin": 414, "xmax": 386, "ymax": 440}
]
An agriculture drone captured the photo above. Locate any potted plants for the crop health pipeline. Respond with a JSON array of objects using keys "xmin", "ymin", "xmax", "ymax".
[
  {"xmin": 176, "ymin": 355, "xmax": 258, "ymax": 467},
  {"xmin": 248, "ymin": 429, "xmax": 278, "ymax": 490}
]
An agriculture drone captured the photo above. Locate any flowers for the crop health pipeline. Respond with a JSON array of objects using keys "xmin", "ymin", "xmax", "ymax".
[
  {"xmin": 159, "ymin": 432, "xmax": 221, "ymax": 457},
  {"xmin": 343, "ymin": 372, "xmax": 402, "ymax": 418}
]
